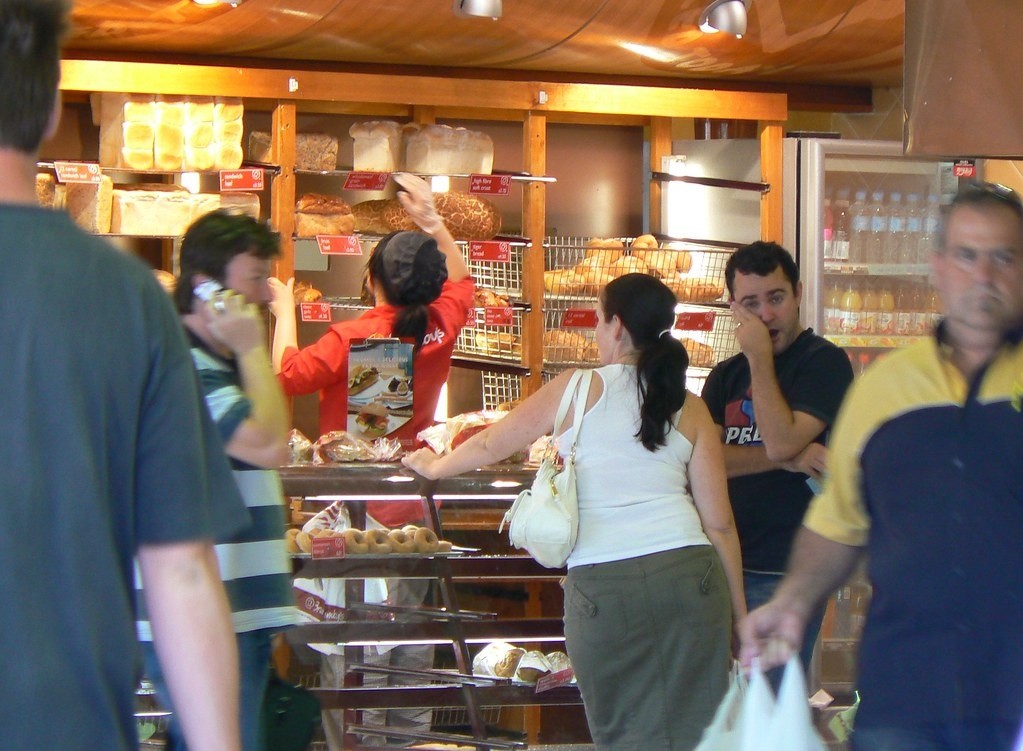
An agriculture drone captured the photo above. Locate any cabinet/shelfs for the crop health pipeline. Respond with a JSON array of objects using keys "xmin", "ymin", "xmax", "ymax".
[
  {"xmin": 272, "ymin": 462, "xmax": 589, "ymax": 749},
  {"xmin": 294, "ymin": 110, "xmax": 534, "ymax": 391},
  {"xmin": 31, "ymin": 84, "xmax": 285, "ymax": 248}
]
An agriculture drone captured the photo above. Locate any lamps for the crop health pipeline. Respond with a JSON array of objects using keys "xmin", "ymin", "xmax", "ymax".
[
  {"xmin": 696, "ymin": 0, "xmax": 749, "ymax": 40},
  {"xmin": 455, "ymin": 1, "xmax": 502, "ymax": 23}
]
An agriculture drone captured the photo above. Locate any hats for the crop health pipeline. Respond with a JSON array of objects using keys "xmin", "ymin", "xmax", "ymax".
[{"xmin": 383, "ymin": 232, "xmax": 448, "ymax": 306}]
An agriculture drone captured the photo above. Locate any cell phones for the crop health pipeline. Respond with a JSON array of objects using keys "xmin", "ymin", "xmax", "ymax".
[{"xmin": 194, "ymin": 278, "xmax": 232, "ymax": 314}]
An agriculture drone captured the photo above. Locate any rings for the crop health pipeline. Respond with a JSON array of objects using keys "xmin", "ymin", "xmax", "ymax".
[{"xmin": 735, "ymin": 322, "xmax": 742, "ymax": 330}]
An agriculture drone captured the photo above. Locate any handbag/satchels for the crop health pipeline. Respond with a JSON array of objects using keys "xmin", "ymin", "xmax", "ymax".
[
  {"xmin": 693, "ymin": 650, "xmax": 827, "ymax": 751},
  {"xmin": 162, "ymin": 669, "xmax": 322, "ymax": 751},
  {"xmin": 499, "ymin": 369, "xmax": 592, "ymax": 568}
]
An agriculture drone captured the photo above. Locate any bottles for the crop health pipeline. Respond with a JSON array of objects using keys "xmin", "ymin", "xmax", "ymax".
[
  {"xmin": 822, "ymin": 276, "xmax": 946, "ymax": 338},
  {"xmin": 822, "ymin": 187, "xmax": 946, "ymax": 265},
  {"xmin": 840, "ymin": 346, "xmax": 888, "ymax": 380}
]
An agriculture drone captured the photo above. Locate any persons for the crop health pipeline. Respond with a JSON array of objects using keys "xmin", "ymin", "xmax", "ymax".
[
  {"xmin": 2, "ymin": 0, "xmax": 240, "ymax": 751},
  {"xmin": 400, "ymin": 275, "xmax": 748, "ymax": 749},
  {"xmin": 702, "ymin": 242, "xmax": 856, "ymax": 708},
  {"xmin": 133, "ymin": 207, "xmax": 296, "ymax": 750},
  {"xmin": 272, "ymin": 168, "xmax": 475, "ymax": 751},
  {"xmin": 738, "ymin": 182, "xmax": 1022, "ymax": 751}
]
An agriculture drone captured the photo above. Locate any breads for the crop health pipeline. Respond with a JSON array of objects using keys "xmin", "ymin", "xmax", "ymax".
[
  {"xmin": 317, "ymin": 430, "xmax": 354, "ymax": 463},
  {"xmin": 37, "ymin": 94, "xmax": 726, "ymax": 366},
  {"xmin": 473, "ymin": 643, "xmax": 551, "ymax": 681}
]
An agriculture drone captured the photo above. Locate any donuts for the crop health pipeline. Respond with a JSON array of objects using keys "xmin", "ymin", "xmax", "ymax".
[{"xmin": 286, "ymin": 525, "xmax": 440, "ymax": 552}]
